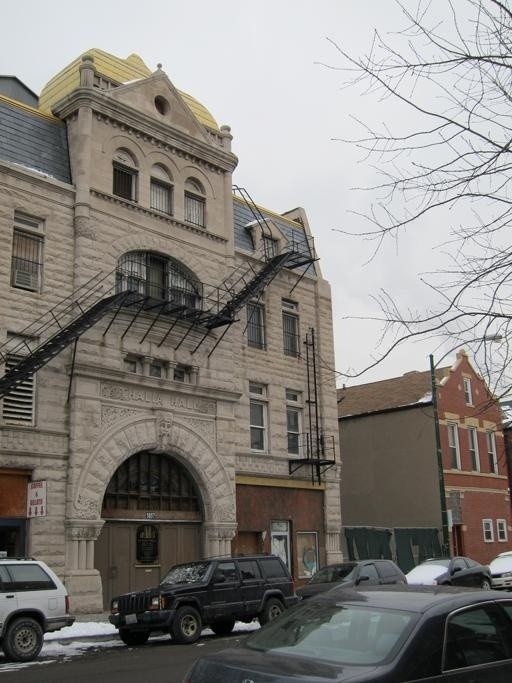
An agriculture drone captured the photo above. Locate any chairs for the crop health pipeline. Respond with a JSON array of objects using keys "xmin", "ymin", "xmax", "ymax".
[{"xmin": 296, "ymin": 615, "xmax": 487, "ymax": 664}]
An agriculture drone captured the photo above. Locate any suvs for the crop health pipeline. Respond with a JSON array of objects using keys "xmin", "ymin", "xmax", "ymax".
[{"xmin": 108, "ymin": 552, "xmax": 300, "ymax": 648}]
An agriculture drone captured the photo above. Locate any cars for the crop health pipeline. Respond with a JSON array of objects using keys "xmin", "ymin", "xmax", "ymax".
[
  {"xmin": 179, "ymin": 582, "xmax": 511, "ymax": 683},
  {"xmin": 1, "ymin": 549, "xmax": 77, "ymax": 663},
  {"xmin": 488, "ymin": 550, "xmax": 512, "ymax": 590},
  {"xmin": 295, "ymin": 559, "xmax": 409, "ymax": 598},
  {"xmin": 404, "ymin": 555, "xmax": 493, "ymax": 592}
]
